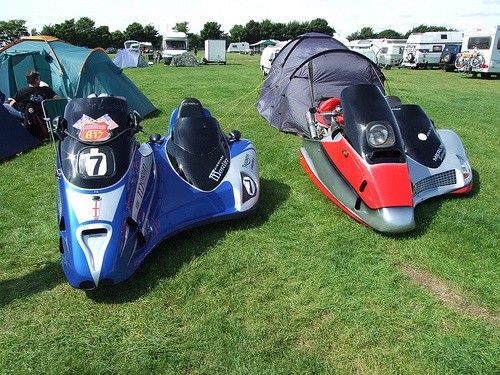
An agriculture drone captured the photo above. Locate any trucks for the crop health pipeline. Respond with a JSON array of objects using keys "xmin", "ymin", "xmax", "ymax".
[
  {"xmin": 349, "ymin": 39, "xmax": 376, "ymax": 60},
  {"xmin": 260, "ymin": 44, "xmax": 286, "ymax": 75},
  {"xmin": 377, "ymin": 38, "xmax": 405, "ymax": 69},
  {"xmin": 454, "ymin": 24, "xmax": 500, "ymax": 79},
  {"xmin": 205, "ymin": 39, "xmax": 226, "ymax": 65},
  {"xmin": 161, "ymin": 32, "xmax": 187, "ymax": 63},
  {"xmin": 227, "ymin": 42, "xmax": 249, "ymax": 53},
  {"xmin": 400, "ymin": 30, "xmax": 463, "ymax": 70}
]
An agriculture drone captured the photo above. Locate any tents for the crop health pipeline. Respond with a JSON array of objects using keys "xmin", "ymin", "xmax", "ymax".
[
  {"xmin": 252, "ymin": 39, "xmax": 276, "ymax": 46},
  {"xmin": 112, "ymin": 49, "xmax": 149, "ymax": 68},
  {"xmin": 255, "ymin": 32, "xmax": 393, "ymax": 139},
  {"xmin": 0, "ymin": 102, "xmax": 43, "ymax": 161},
  {"xmin": 169, "ymin": 52, "xmax": 198, "ymax": 67}
]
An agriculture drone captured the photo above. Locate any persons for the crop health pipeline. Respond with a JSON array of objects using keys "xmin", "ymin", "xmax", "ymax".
[
  {"xmin": 157, "ymin": 52, "xmax": 162, "ymax": 63},
  {"xmin": 0, "ymin": 35, "xmax": 155, "ymax": 144},
  {"xmin": 3, "ymin": 98, "xmax": 26, "ymax": 127},
  {"xmin": 194, "ymin": 48, "xmax": 197, "ymax": 56},
  {"xmin": 12, "ymin": 70, "xmax": 62, "ymax": 135}
]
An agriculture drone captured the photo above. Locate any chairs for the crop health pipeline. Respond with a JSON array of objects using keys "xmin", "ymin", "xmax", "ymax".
[
  {"xmin": 41, "ymin": 99, "xmax": 68, "ymax": 154},
  {"xmin": 177, "ymin": 98, "xmax": 204, "ymax": 118},
  {"xmin": 385, "ymin": 96, "xmax": 401, "ymax": 106},
  {"xmin": 148, "ymin": 54, "xmax": 155, "ymax": 62}
]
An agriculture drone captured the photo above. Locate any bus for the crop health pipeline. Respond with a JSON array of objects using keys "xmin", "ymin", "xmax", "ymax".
[{"xmin": 124, "ymin": 41, "xmax": 154, "ymax": 60}]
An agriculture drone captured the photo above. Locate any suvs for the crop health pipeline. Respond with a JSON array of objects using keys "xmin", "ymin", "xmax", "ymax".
[{"xmin": 437, "ymin": 43, "xmax": 462, "ymax": 73}]
